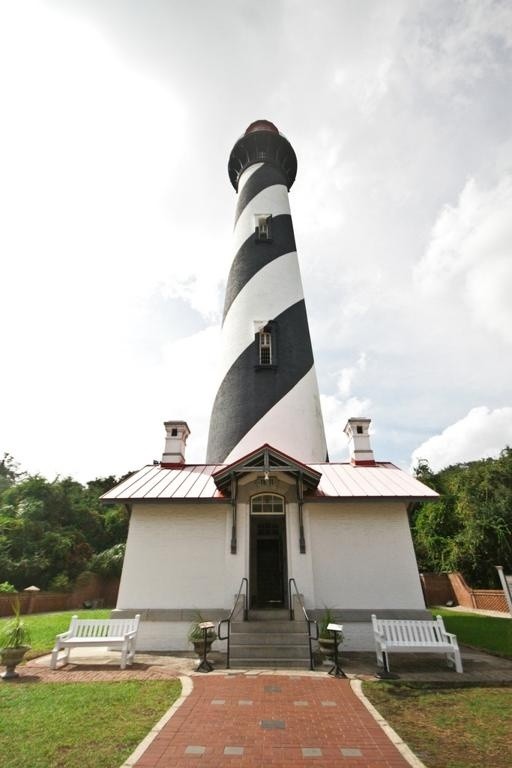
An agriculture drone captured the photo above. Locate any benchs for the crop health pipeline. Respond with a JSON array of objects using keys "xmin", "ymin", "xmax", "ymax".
[
  {"xmin": 368, "ymin": 611, "xmax": 464, "ymax": 676},
  {"xmin": 50, "ymin": 614, "xmax": 143, "ymax": 669}
]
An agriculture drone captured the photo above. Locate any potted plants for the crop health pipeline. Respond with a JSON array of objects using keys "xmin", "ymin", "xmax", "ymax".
[
  {"xmin": 0, "ymin": 594, "xmax": 33, "ymax": 681},
  {"xmin": 317, "ymin": 605, "xmax": 346, "ymax": 668},
  {"xmin": 187, "ymin": 611, "xmax": 220, "ymax": 666}
]
